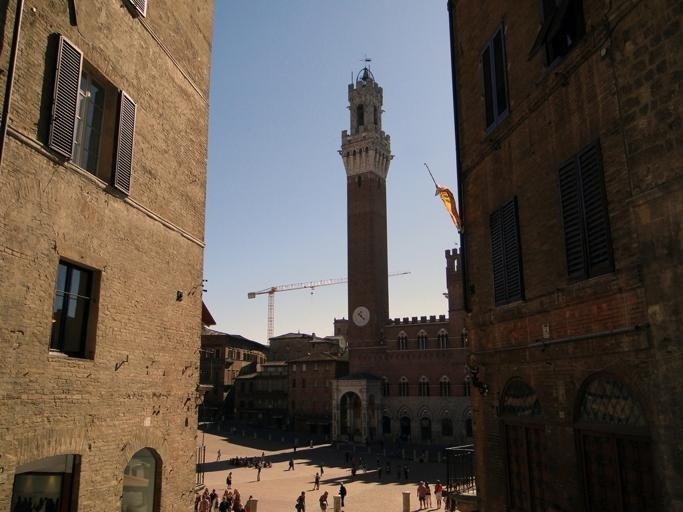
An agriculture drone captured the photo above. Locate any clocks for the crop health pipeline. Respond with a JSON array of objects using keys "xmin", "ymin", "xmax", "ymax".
[{"xmin": 352, "ymin": 306, "xmax": 370, "ymax": 327}]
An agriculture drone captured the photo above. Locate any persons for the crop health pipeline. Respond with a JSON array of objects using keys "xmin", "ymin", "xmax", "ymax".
[
  {"xmin": 343, "ymin": 450, "xmax": 409, "ymax": 482},
  {"xmin": 296, "ymin": 491, "xmax": 306, "ymax": 511},
  {"xmin": 193, "ymin": 487, "xmax": 254, "ymax": 512},
  {"xmin": 424, "ymin": 481, "xmax": 434, "ymax": 508},
  {"xmin": 313, "ymin": 472, "xmax": 321, "ymax": 490},
  {"xmin": 339, "ymin": 482, "xmax": 346, "ymax": 507},
  {"xmin": 287, "ymin": 458, "xmax": 294, "ymax": 471},
  {"xmin": 433, "ymin": 480, "xmax": 443, "ymax": 510},
  {"xmin": 319, "ymin": 462, "xmax": 324, "ymax": 476},
  {"xmin": 216, "ymin": 449, "xmax": 222, "ymax": 462},
  {"xmin": 225, "ymin": 472, "xmax": 233, "ymax": 490},
  {"xmin": 229, "ymin": 452, "xmax": 273, "ymax": 482},
  {"xmin": 417, "ymin": 481, "xmax": 427, "ymax": 509},
  {"xmin": 319, "ymin": 491, "xmax": 329, "ymax": 512}
]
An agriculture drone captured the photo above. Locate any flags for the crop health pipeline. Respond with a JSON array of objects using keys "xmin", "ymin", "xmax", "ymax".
[{"xmin": 434, "ymin": 184, "xmax": 462, "ymax": 227}]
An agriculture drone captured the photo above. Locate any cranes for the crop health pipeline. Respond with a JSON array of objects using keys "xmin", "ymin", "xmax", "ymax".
[{"xmin": 245, "ymin": 269, "xmax": 413, "ymax": 343}]
